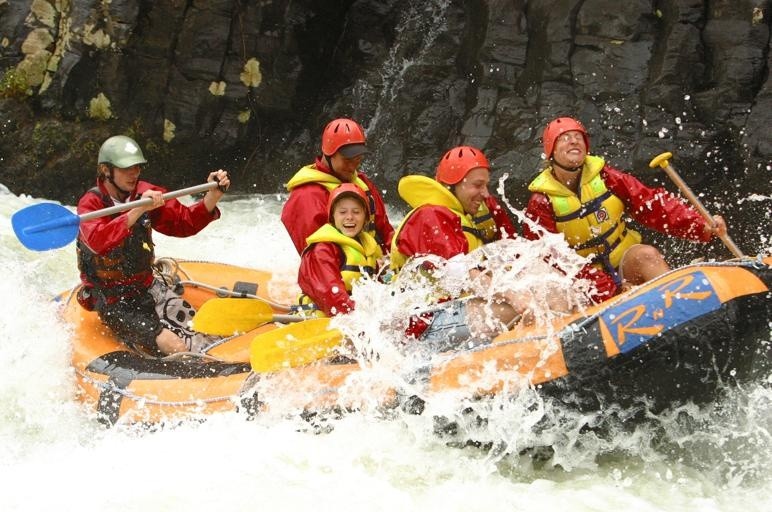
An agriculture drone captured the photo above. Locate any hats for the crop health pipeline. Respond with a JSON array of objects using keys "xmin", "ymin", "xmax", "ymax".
[{"xmin": 338, "ymin": 143, "xmax": 372, "ymax": 160}]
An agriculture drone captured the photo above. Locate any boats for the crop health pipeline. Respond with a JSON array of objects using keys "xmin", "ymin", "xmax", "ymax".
[{"xmin": 48, "ymin": 248, "xmax": 772, "ymax": 432}]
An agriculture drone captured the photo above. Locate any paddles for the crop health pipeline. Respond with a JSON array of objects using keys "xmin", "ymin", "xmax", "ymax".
[
  {"xmin": 11, "ymin": 175, "xmax": 227, "ymax": 252},
  {"xmin": 249, "ymin": 295, "xmax": 480, "ymax": 371},
  {"xmin": 192, "ymin": 297, "xmax": 321, "ymax": 339}
]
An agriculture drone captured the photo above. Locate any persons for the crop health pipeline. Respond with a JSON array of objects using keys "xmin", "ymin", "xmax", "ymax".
[
  {"xmin": 389, "ymin": 147, "xmax": 531, "ymax": 350},
  {"xmin": 524, "ymin": 117, "xmax": 726, "ymax": 306},
  {"xmin": 280, "ymin": 119, "xmax": 395, "ymax": 283},
  {"xmin": 297, "ymin": 183, "xmax": 384, "ymax": 318},
  {"xmin": 76, "ymin": 135, "xmax": 230, "ymax": 363},
  {"xmin": 466, "ymin": 196, "xmax": 518, "ymax": 243}
]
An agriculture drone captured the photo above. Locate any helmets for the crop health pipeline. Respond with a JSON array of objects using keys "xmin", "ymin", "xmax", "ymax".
[
  {"xmin": 321, "ymin": 118, "xmax": 365, "ymax": 156},
  {"xmin": 543, "ymin": 117, "xmax": 589, "ymax": 160},
  {"xmin": 328, "ymin": 182, "xmax": 370, "ymax": 223},
  {"xmin": 97, "ymin": 135, "xmax": 147, "ymax": 169},
  {"xmin": 436, "ymin": 146, "xmax": 489, "ymax": 185}
]
